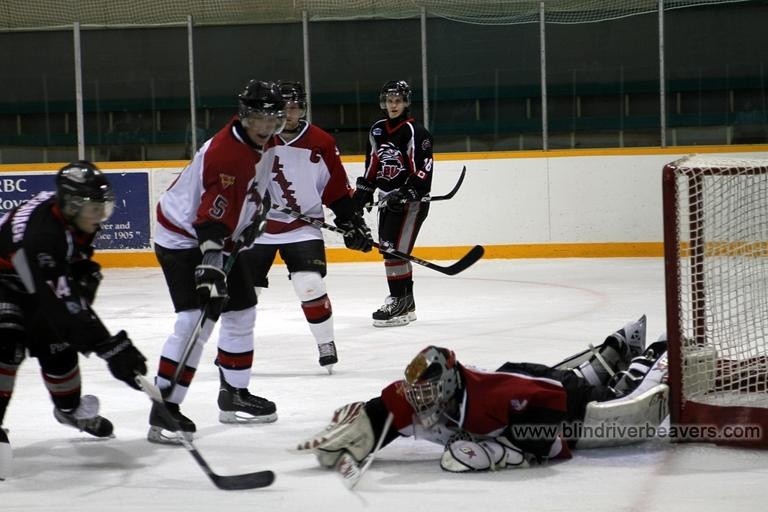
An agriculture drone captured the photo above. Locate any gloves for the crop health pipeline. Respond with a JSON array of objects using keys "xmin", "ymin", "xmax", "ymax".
[
  {"xmin": 334, "ymin": 214, "xmax": 373, "ymax": 252},
  {"xmin": 94, "ymin": 330, "xmax": 147, "ymax": 391},
  {"xmin": 355, "ymin": 174, "xmax": 376, "ymax": 216},
  {"xmin": 69, "ymin": 257, "xmax": 103, "ymax": 302},
  {"xmin": 192, "ymin": 264, "xmax": 229, "ymax": 321},
  {"xmin": 386, "ymin": 186, "xmax": 412, "ymax": 215}
]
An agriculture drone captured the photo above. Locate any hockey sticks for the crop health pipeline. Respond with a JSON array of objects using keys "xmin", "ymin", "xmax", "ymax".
[
  {"xmin": 337, "ymin": 412, "xmax": 396, "ymax": 490},
  {"xmin": 135, "ymin": 373, "xmax": 274, "ymax": 491},
  {"xmin": 159, "ymin": 247, "xmax": 237, "ymax": 399},
  {"xmin": 269, "ymin": 201, "xmax": 484, "ymax": 275},
  {"xmin": 366, "ymin": 165, "xmax": 466, "ymax": 208}
]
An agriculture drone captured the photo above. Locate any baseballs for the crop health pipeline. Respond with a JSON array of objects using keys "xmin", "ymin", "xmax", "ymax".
[{"xmin": 81, "ymin": 395, "xmax": 100, "ymax": 418}]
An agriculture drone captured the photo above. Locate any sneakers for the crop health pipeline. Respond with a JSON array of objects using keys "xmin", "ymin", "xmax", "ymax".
[
  {"xmin": 317, "ymin": 340, "xmax": 338, "ymax": 366},
  {"xmin": 54, "ymin": 394, "xmax": 113, "ymax": 437},
  {"xmin": 372, "ymin": 296, "xmax": 416, "ymax": 321},
  {"xmin": 149, "ymin": 400, "xmax": 197, "ymax": 433},
  {"xmin": 217, "ymin": 389, "xmax": 277, "ymax": 416}
]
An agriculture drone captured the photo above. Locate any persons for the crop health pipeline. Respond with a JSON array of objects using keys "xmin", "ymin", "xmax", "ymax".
[
  {"xmin": 349, "ymin": 80, "xmax": 433, "ymax": 320},
  {"xmin": 147, "ymin": 78, "xmax": 288, "ymax": 432},
  {"xmin": 253, "ymin": 77, "xmax": 374, "ymax": 365},
  {"xmin": 0, "ymin": 161, "xmax": 146, "ymax": 443},
  {"xmin": 290, "ymin": 317, "xmax": 767, "ymax": 474}
]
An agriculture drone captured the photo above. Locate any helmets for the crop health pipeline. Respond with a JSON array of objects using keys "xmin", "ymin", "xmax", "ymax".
[
  {"xmin": 379, "ymin": 79, "xmax": 414, "ymax": 109},
  {"xmin": 56, "ymin": 160, "xmax": 115, "ymax": 213},
  {"xmin": 235, "ymin": 77, "xmax": 287, "ymax": 123},
  {"xmin": 279, "ymin": 82, "xmax": 308, "ymax": 109},
  {"xmin": 400, "ymin": 344, "xmax": 462, "ymax": 414}
]
